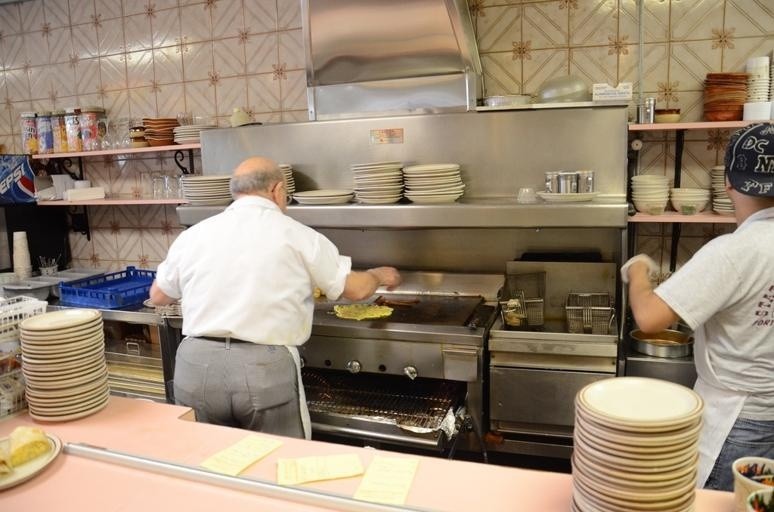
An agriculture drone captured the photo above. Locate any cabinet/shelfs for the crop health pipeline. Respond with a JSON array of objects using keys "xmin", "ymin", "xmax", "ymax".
[
  {"xmin": 627, "ymin": 120, "xmax": 774, "ymax": 224},
  {"xmin": 31, "ymin": 142, "xmax": 201, "ymax": 206}
]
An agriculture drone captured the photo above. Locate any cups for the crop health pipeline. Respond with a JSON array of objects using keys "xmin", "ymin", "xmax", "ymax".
[
  {"xmin": 11, "ymin": 231, "xmax": 33, "ymax": 280},
  {"xmin": 731, "ymin": 456, "xmax": 774, "ymax": 512},
  {"xmin": 518, "ymin": 187, "xmax": 538, "ymax": 203}
]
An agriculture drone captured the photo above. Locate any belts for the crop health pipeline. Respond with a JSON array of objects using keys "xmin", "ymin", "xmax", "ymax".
[{"xmin": 194, "ymin": 336, "xmax": 250, "ymax": 345}]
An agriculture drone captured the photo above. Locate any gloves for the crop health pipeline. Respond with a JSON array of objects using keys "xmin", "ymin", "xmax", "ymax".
[{"xmin": 620, "ymin": 252, "xmax": 656, "ymax": 283}]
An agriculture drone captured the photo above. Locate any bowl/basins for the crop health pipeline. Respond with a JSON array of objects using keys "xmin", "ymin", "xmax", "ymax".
[
  {"xmin": 538, "ymin": 74, "xmax": 589, "ymax": 103},
  {"xmin": 631, "ymin": 173, "xmax": 671, "ymax": 214},
  {"xmin": 702, "ymin": 53, "xmax": 761, "ymax": 121},
  {"xmin": 655, "ymin": 108, "xmax": 681, "ymax": 123},
  {"xmin": 129, "ymin": 126, "xmax": 148, "ymax": 147},
  {"xmin": 710, "ymin": 164, "xmax": 735, "ymax": 217},
  {"xmin": 671, "ymin": 186, "xmax": 710, "ymax": 213}
]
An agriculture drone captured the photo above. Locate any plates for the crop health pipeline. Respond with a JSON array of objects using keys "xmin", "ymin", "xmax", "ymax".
[
  {"xmin": 142, "ymin": 116, "xmax": 219, "ymax": 146},
  {"xmin": 537, "ymin": 189, "xmax": 596, "ymax": 201},
  {"xmin": 180, "ymin": 173, "xmax": 235, "ymax": 206},
  {"xmin": 2, "ymin": 430, "xmax": 62, "ymax": 493},
  {"xmin": 568, "ymin": 376, "xmax": 704, "ymax": 512},
  {"xmin": 482, "ymin": 93, "xmax": 533, "ymax": 106},
  {"xmin": 351, "ymin": 159, "xmax": 467, "ymax": 203},
  {"xmin": 18, "ymin": 307, "xmax": 112, "ymax": 423},
  {"xmin": 277, "ymin": 163, "xmax": 355, "ymax": 204}
]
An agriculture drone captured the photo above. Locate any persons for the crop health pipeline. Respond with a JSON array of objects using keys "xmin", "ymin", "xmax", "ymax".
[
  {"xmin": 620, "ymin": 123, "xmax": 774, "ymax": 491},
  {"xmin": 148, "ymin": 157, "xmax": 402, "ymax": 443}
]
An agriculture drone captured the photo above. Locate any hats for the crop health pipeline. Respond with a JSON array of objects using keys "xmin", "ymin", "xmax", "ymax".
[{"xmin": 724, "ymin": 121, "xmax": 774, "ymax": 198}]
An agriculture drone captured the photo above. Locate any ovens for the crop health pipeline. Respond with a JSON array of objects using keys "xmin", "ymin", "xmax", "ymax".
[{"xmin": 294, "ymin": 336, "xmax": 482, "ymax": 459}]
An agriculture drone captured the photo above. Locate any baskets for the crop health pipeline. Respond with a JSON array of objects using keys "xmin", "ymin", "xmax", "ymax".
[{"xmin": 59, "ymin": 265, "xmax": 158, "ymax": 309}]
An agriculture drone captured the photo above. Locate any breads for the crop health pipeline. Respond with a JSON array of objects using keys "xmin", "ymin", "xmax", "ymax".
[{"xmin": 7, "ymin": 424, "xmax": 52, "ymax": 468}]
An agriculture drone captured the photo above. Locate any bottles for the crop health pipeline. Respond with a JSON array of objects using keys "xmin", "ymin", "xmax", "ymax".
[{"xmin": 545, "ymin": 169, "xmax": 595, "ymax": 194}]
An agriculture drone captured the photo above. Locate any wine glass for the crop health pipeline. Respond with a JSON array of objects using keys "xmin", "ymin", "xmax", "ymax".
[{"xmin": 89, "ymin": 116, "xmax": 133, "ymax": 150}]
